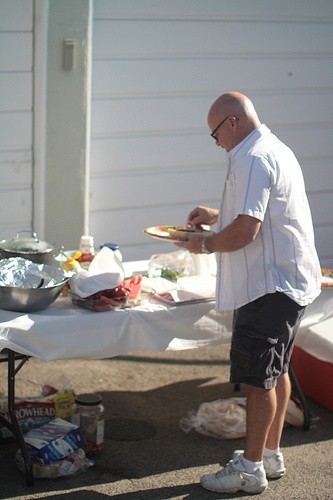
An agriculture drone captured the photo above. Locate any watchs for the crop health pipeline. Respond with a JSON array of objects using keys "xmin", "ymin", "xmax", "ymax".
[{"xmin": 202, "ymin": 239, "xmax": 212, "ymax": 254}]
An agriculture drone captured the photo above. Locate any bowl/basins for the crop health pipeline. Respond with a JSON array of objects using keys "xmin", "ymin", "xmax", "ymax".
[{"xmin": 67, "ymin": 284, "xmax": 157, "ymax": 312}]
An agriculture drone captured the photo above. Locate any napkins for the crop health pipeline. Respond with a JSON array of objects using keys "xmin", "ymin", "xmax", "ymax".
[{"xmin": 69, "ymin": 247, "xmax": 125, "ymax": 299}]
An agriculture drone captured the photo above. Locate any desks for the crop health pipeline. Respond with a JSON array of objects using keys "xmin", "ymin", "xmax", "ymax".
[{"xmin": 0, "ymin": 253, "xmax": 333, "ymax": 486}]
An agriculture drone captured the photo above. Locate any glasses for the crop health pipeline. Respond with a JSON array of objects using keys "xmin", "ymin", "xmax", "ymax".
[{"xmin": 210, "ymin": 115, "xmax": 239, "ymax": 141}]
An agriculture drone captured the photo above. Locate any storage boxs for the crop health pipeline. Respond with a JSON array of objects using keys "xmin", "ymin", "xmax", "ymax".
[
  {"xmin": 290, "ymin": 286, "xmax": 333, "ymax": 412},
  {"xmin": 19, "ymin": 417, "xmax": 83, "ymax": 466}
]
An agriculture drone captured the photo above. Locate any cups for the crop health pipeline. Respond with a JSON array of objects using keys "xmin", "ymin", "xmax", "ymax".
[{"xmin": 55, "ymin": 250, "xmax": 79, "ymax": 297}]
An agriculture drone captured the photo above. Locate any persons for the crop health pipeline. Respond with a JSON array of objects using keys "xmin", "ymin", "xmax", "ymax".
[{"xmin": 175, "ymin": 91, "xmax": 323, "ymax": 494}]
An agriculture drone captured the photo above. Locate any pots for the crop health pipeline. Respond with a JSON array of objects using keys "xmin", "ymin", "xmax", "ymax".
[
  {"xmin": 0, "ymin": 230, "xmax": 57, "ymax": 265},
  {"xmin": 0, "ymin": 271, "xmax": 79, "ymax": 312}
]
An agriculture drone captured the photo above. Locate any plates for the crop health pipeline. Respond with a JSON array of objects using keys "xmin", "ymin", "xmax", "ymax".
[{"xmin": 143, "ymin": 224, "xmax": 211, "ymax": 242}]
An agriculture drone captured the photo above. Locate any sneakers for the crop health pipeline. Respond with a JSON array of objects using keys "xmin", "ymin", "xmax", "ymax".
[
  {"xmin": 199, "ymin": 457, "xmax": 268, "ymax": 493},
  {"xmin": 231, "ymin": 450, "xmax": 287, "ymax": 478}
]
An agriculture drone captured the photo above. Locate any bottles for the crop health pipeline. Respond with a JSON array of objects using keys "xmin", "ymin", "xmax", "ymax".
[
  {"xmin": 100, "ymin": 242, "xmax": 122, "ymax": 263},
  {"xmin": 76, "ymin": 235, "xmax": 96, "ymax": 271},
  {"xmin": 70, "ymin": 393, "xmax": 106, "ymax": 454}
]
